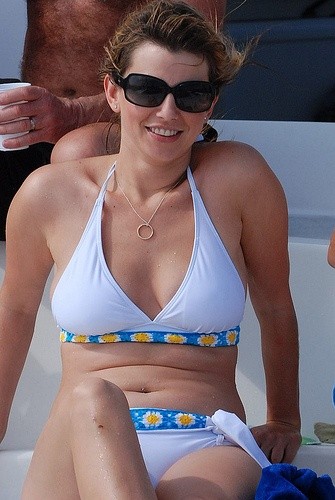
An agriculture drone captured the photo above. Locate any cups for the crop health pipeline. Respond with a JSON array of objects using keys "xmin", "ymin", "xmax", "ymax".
[{"xmin": 0, "ymin": 83, "xmax": 31, "ymax": 151}]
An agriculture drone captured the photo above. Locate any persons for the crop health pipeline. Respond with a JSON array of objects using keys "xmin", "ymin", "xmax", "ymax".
[
  {"xmin": 0, "ymin": 0, "xmax": 304, "ymax": 500},
  {"xmin": 0, "ymin": 0, "xmax": 227, "ymax": 241}
]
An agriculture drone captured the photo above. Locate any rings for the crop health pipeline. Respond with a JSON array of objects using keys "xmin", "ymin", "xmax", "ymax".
[{"xmin": 29, "ymin": 116, "xmax": 37, "ymax": 131}]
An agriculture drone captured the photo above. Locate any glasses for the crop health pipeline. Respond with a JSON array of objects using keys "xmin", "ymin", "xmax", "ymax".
[{"xmin": 112, "ymin": 70, "xmax": 218, "ymax": 113}]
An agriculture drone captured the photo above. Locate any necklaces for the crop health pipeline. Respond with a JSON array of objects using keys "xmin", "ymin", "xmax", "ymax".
[{"xmin": 113, "ymin": 155, "xmax": 187, "ymax": 241}]
